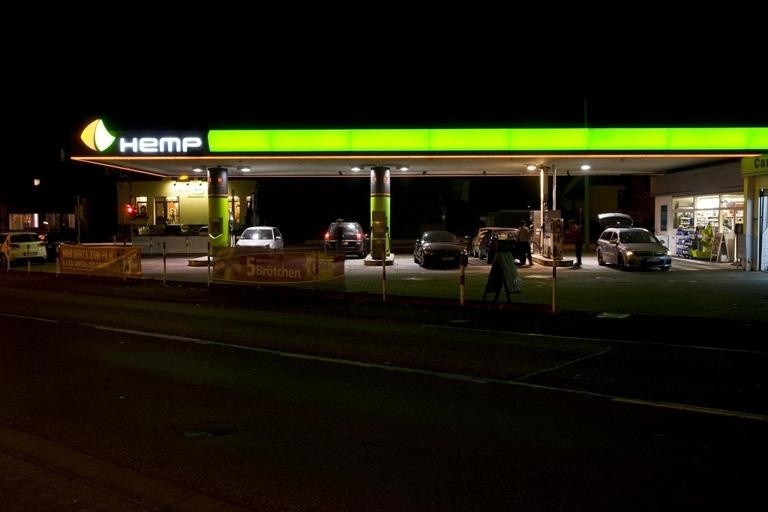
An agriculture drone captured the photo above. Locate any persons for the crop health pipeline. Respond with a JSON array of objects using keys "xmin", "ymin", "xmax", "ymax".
[
  {"xmin": 564, "ymin": 219, "xmax": 585, "ymax": 266},
  {"xmin": 136, "ymin": 206, "xmax": 147, "ymax": 218},
  {"xmin": 724, "ymin": 219, "xmax": 732, "ymax": 230},
  {"xmin": 516, "ymin": 220, "xmax": 534, "ymax": 266},
  {"xmin": 333, "ymin": 217, "xmax": 345, "ymax": 256}
]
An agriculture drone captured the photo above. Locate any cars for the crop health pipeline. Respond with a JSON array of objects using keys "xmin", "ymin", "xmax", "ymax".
[
  {"xmin": 236, "ymin": 226, "xmax": 284, "ymax": 249},
  {"xmin": 0, "ymin": 231, "xmax": 48, "ymax": 266},
  {"xmin": 323, "ymin": 222, "xmax": 368, "ymax": 258},
  {"xmin": 596, "ymin": 212, "xmax": 671, "ymax": 272},
  {"xmin": 413, "ymin": 226, "xmax": 524, "ymax": 268}
]
